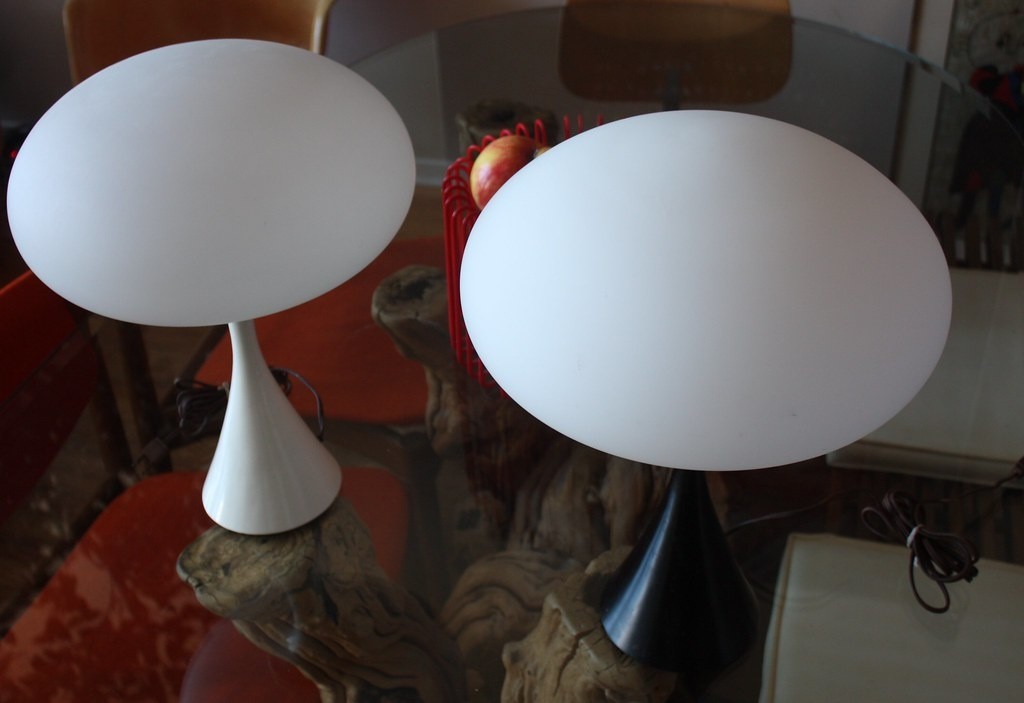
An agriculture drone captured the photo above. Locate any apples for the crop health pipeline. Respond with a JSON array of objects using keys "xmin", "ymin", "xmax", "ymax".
[{"xmin": 469, "ymin": 135, "xmax": 553, "ymax": 211}]
